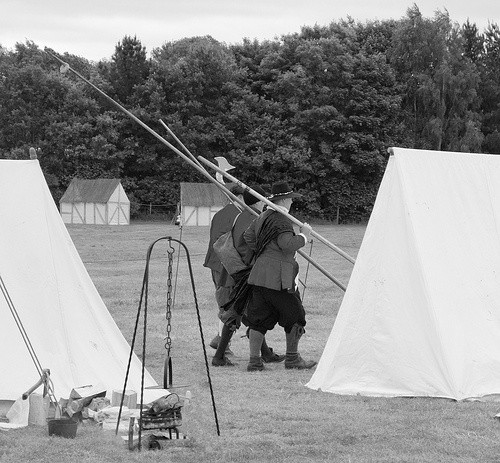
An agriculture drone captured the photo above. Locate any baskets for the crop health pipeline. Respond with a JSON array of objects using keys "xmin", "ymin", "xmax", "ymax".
[{"xmin": 142, "ymin": 394, "xmax": 183, "ymax": 430}]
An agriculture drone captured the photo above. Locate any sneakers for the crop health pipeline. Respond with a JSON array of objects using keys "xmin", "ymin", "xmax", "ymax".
[
  {"xmin": 210, "ymin": 333, "xmax": 234, "ymax": 357},
  {"xmin": 248, "ymin": 356, "xmax": 274, "ymax": 372},
  {"xmin": 284, "ymin": 351, "xmax": 318, "ymax": 370}
]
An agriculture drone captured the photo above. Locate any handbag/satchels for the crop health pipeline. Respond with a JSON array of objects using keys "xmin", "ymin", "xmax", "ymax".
[{"xmin": 212, "ymin": 231, "xmax": 247, "ymax": 281}]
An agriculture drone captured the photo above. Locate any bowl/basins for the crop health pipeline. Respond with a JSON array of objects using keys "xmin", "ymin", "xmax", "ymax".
[{"xmin": 48, "ymin": 419, "xmax": 78, "ymax": 439}]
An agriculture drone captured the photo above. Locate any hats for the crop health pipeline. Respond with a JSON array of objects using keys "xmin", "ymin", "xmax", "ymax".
[
  {"xmin": 267, "ymin": 180, "xmax": 303, "ymax": 202},
  {"xmin": 229, "ymin": 186, "xmax": 246, "ymax": 194},
  {"xmin": 243, "ymin": 185, "xmax": 271, "ymax": 205}
]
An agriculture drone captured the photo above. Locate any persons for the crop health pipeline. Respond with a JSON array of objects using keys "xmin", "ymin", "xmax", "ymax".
[
  {"xmin": 203, "ymin": 185, "xmax": 286, "ymax": 368},
  {"xmin": 244, "ymin": 182, "xmax": 317, "ymax": 373}
]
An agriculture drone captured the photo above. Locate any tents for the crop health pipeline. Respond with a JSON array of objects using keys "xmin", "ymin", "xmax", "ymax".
[
  {"xmin": 0, "ymin": 159, "xmax": 172, "ymax": 405},
  {"xmin": 306, "ymin": 147, "xmax": 500, "ymax": 402}
]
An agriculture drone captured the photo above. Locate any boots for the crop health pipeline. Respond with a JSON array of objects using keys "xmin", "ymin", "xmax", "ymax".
[
  {"xmin": 210, "ymin": 322, "xmax": 241, "ymax": 366},
  {"xmin": 246, "ymin": 327, "xmax": 286, "ymax": 363}
]
